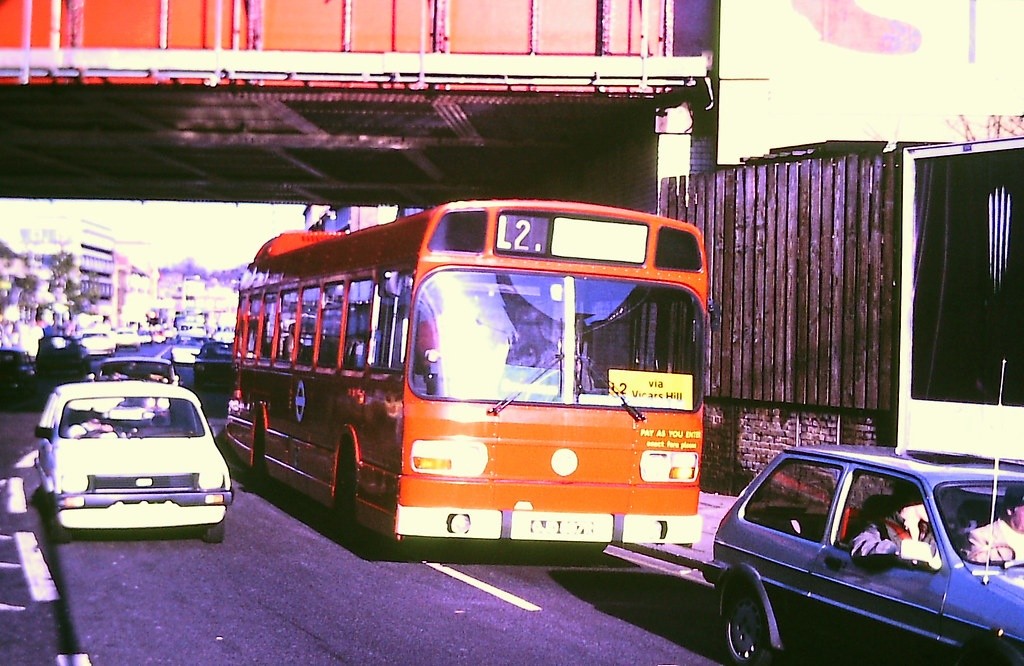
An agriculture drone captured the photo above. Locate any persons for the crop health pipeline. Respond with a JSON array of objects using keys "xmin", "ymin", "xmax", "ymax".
[
  {"xmin": 954, "ymin": 483, "xmax": 1024, "ymax": 564},
  {"xmin": 848, "ymin": 486, "xmax": 946, "ymax": 561}
]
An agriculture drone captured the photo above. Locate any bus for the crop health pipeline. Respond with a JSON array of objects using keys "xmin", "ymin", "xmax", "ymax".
[{"xmin": 220, "ymin": 199, "xmax": 706, "ymax": 562}]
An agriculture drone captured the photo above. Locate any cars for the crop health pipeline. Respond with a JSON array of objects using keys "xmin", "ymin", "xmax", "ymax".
[
  {"xmin": 91, "ymin": 353, "xmax": 184, "ymax": 394},
  {"xmin": 26, "ymin": 377, "xmax": 233, "ymax": 545},
  {"xmin": 700, "ymin": 445, "xmax": 1024, "ymax": 666},
  {"xmin": 136, "ymin": 314, "xmax": 236, "ymax": 365},
  {"xmin": 1, "ymin": 346, "xmax": 39, "ymax": 400},
  {"xmin": 115, "ymin": 328, "xmax": 141, "ymax": 350},
  {"xmin": 80, "ymin": 331, "xmax": 116, "ymax": 356},
  {"xmin": 191, "ymin": 340, "xmax": 233, "ymax": 381},
  {"xmin": 34, "ymin": 332, "xmax": 88, "ymax": 377}
]
